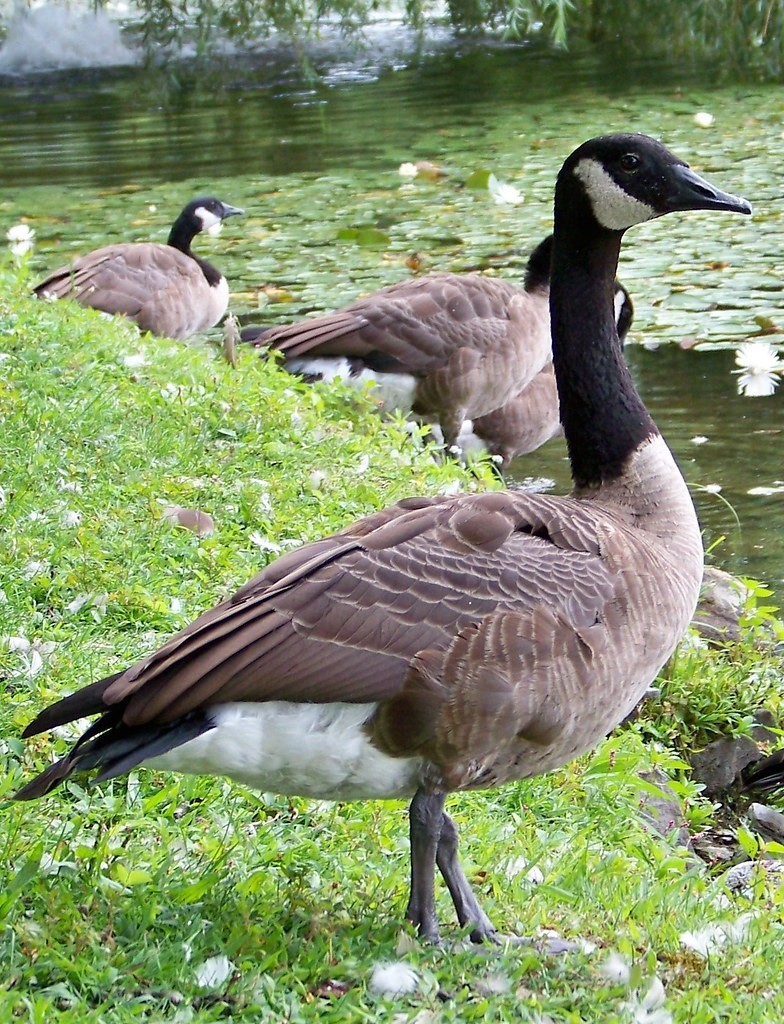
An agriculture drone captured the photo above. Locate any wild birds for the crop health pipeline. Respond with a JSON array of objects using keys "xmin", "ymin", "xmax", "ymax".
[{"xmin": 13, "ymin": 133, "xmax": 752, "ymax": 998}]
